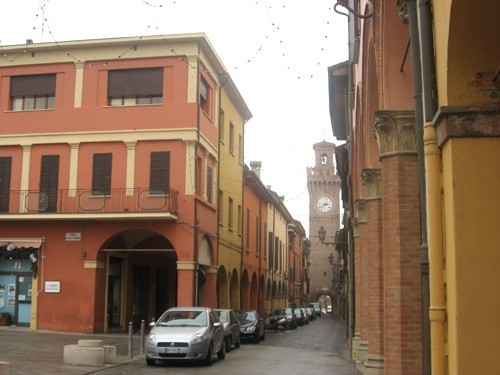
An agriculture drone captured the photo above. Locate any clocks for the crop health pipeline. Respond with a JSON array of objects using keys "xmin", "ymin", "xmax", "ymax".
[{"xmin": 317, "ymin": 197, "xmax": 332, "ymax": 212}]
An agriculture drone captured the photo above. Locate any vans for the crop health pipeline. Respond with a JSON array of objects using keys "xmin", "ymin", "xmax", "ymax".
[{"xmin": 310, "ymin": 303, "xmax": 322, "ymax": 314}]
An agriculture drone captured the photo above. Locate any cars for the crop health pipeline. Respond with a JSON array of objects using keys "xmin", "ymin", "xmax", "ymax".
[
  {"xmin": 144, "ymin": 307, "xmax": 225, "ymax": 365},
  {"xmin": 294, "ymin": 308, "xmax": 315, "ymax": 325},
  {"xmin": 269, "ymin": 308, "xmax": 297, "ymax": 329},
  {"xmin": 233, "ymin": 309, "xmax": 265, "ymax": 343},
  {"xmin": 214, "ymin": 309, "xmax": 240, "ymax": 352}
]
